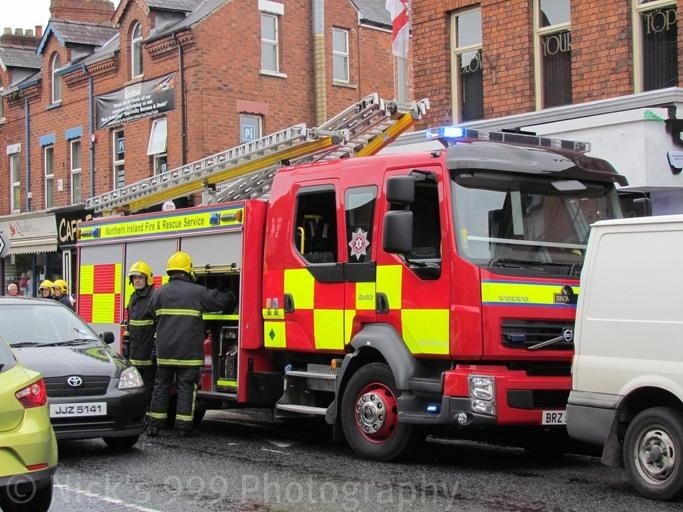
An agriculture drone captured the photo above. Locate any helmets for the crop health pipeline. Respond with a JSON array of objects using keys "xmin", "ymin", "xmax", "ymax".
[
  {"xmin": 166, "ymin": 251, "xmax": 193, "ymax": 277},
  {"xmin": 39, "ymin": 279, "xmax": 68, "ymax": 297},
  {"xmin": 128, "ymin": 260, "xmax": 154, "ymax": 285}
]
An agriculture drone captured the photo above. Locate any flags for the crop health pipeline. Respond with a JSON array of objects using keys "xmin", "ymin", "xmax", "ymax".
[{"xmin": 386, "ymin": 0, "xmax": 411, "ymax": 57}]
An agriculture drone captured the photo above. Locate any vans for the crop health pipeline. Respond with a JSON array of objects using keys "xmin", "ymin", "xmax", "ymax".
[{"xmin": 563, "ymin": 213, "xmax": 683, "ymax": 502}]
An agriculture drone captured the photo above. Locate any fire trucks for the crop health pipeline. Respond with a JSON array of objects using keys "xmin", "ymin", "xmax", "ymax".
[{"xmin": 75, "ymin": 91, "xmax": 630, "ymax": 463}]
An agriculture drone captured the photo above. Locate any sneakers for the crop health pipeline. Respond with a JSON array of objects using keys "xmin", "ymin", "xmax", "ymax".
[{"xmin": 143, "ymin": 423, "xmax": 193, "ymax": 437}]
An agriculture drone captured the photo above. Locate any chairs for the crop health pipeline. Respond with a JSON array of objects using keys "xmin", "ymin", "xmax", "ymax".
[{"xmin": 303, "ymin": 216, "xmax": 515, "ymax": 267}]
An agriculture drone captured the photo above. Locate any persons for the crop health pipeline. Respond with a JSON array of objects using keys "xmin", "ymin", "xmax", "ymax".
[
  {"xmin": 8, "ymin": 284, "xmax": 19, "ymax": 297},
  {"xmin": 39, "ymin": 279, "xmax": 75, "ymax": 313},
  {"xmin": 120, "ymin": 251, "xmax": 236, "ymax": 436}
]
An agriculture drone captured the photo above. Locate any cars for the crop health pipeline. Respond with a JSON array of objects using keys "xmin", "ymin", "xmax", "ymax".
[
  {"xmin": 0, "ymin": 332, "xmax": 59, "ymax": 511},
  {"xmin": 1, "ymin": 295, "xmax": 151, "ymax": 452}
]
